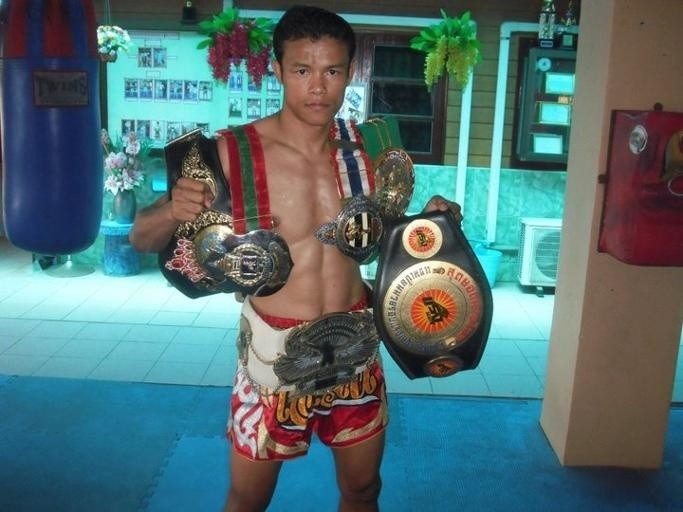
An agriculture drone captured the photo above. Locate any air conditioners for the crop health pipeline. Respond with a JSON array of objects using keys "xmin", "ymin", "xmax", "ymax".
[{"xmin": 516, "ymin": 217, "xmax": 562, "ymax": 287}]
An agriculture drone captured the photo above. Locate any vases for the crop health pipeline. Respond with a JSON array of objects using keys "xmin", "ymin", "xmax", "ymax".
[
  {"xmin": 99, "ymin": 51, "xmax": 116, "ymax": 63},
  {"xmin": 112, "ymin": 188, "xmax": 136, "ymax": 224}
]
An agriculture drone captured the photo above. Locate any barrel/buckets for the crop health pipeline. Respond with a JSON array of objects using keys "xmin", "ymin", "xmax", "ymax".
[
  {"xmin": 475, "ymin": 247, "xmax": 502, "ymax": 287},
  {"xmin": 104, "ymin": 235, "xmax": 141, "ymax": 278}
]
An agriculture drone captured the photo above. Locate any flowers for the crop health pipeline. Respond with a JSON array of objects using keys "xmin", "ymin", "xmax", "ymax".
[
  {"xmin": 100, "ymin": 127, "xmax": 163, "ymax": 197},
  {"xmin": 96, "ymin": 24, "xmax": 130, "ymax": 54},
  {"xmin": 195, "ymin": 5, "xmax": 274, "ymax": 90},
  {"xmin": 407, "ymin": 7, "xmax": 484, "ymax": 92}
]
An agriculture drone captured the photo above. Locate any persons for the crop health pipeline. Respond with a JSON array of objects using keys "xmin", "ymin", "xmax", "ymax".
[{"xmin": 125, "ymin": 3, "xmax": 466, "ymax": 512}]
